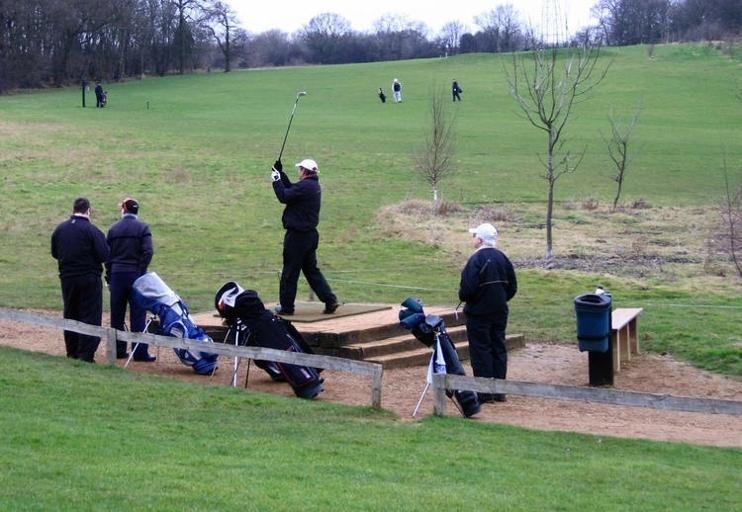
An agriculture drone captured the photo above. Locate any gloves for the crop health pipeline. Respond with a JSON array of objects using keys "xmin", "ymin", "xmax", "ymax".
[
  {"xmin": 270, "ymin": 165, "xmax": 281, "ymax": 183},
  {"xmin": 272, "ymin": 160, "xmax": 284, "ymax": 171}
]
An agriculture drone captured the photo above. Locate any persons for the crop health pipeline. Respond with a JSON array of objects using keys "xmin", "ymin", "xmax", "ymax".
[
  {"xmin": 51, "ymin": 198, "xmax": 110, "ymax": 363},
  {"xmin": 391, "ymin": 79, "xmax": 402, "ymax": 103},
  {"xmin": 270, "ymin": 160, "xmax": 341, "ymax": 315},
  {"xmin": 451, "ymin": 81, "xmax": 462, "ymax": 101},
  {"xmin": 102, "ymin": 199, "xmax": 156, "ymax": 363},
  {"xmin": 457, "ymin": 223, "xmax": 519, "ymax": 400},
  {"xmin": 95, "ymin": 81, "xmax": 106, "ymax": 108}
]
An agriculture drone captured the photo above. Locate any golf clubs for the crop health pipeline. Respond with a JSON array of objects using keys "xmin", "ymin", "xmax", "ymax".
[
  {"xmin": 278, "ymin": 92, "xmax": 306, "ymax": 161},
  {"xmin": 454, "ymin": 260, "xmax": 492, "ymax": 320}
]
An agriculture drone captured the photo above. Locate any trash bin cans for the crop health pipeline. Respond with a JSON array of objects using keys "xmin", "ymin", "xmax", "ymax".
[{"xmin": 575, "ymin": 294, "xmax": 611, "ymax": 353}]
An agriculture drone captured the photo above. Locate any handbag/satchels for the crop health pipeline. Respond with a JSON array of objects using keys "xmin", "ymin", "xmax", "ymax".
[
  {"xmin": 213, "ymin": 282, "xmax": 289, "ymax": 384},
  {"xmin": 235, "ymin": 289, "xmax": 326, "ymax": 401},
  {"xmin": 421, "ymin": 311, "xmax": 482, "ymax": 419},
  {"xmin": 131, "ymin": 270, "xmax": 218, "ymax": 377}
]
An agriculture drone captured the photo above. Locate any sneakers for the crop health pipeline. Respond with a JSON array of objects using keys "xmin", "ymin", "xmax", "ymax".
[
  {"xmin": 134, "ymin": 354, "xmax": 158, "ymax": 362},
  {"xmin": 118, "ymin": 351, "xmax": 130, "ymax": 358},
  {"xmin": 321, "ymin": 301, "xmax": 340, "ymax": 315},
  {"xmin": 477, "ymin": 392, "xmax": 494, "ymax": 405},
  {"xmin": 495, "ymin": 393, "xmax": 507, "ymax": 402},
  {"xmin": 274, "ymin": 303, "xmax": 295, "ymax": 315}
]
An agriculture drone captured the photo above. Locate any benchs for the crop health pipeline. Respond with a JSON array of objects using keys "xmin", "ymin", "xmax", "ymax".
[{"xmin": 608, "ymin": 302, "xmax": 647, "ymax": 374}]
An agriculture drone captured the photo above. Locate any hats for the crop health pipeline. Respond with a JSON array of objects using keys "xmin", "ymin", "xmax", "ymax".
[
  {"xmin": 294, "ymin": 159, "xmax": 320, "ymax": 174},
  {"xmin": 468, "ymin": 222, "xmax": 498, "ymax": 246},
  {"xmin": 117, "ymin": 197, "xmax": 140, "ymax": 215}
]
